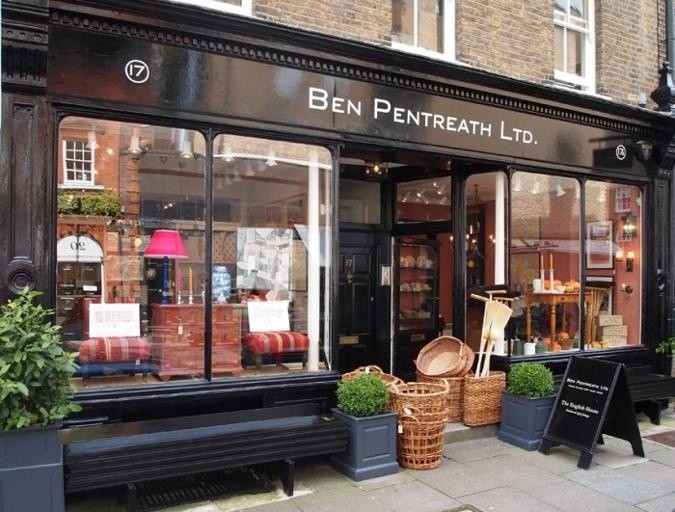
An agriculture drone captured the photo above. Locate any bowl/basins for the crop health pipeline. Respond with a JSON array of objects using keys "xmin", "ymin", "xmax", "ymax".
[
  {"xmin": 401, "ymin": 311, "xmax": 417, "ymax": 319},
  {"xmin": 556, "ymin": 286, "xmax": 566, "ymax": 293}
]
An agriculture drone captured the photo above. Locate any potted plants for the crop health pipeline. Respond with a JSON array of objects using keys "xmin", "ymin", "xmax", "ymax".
[
  {"xmin": 496, "ymin": 359, "xmax": 558, "ymax": 452},
  {"xmin": 331, "ymin": 372, "xmax": 401, "ymax": 483},
  {"xmin": 0, "ymin": 282, "xmax": 85, "ymax": 511}
]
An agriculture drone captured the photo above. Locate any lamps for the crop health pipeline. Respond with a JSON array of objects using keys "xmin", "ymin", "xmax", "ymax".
[
  {"xmin": 142, "ymin": 229, "xmax": 190, "ymax": 303},
  {"xmin": 514, "ymin": 173, "xmax": 568, "ymax": 198},
  {"xmin": 400, "ymin": 184, "xmax": 447, "ymax": 207},
  {"xmin": 85, "ymin": 120, "xmax": 198, "ymax": 161},
  {"xmin": 219, "ymin": 136, "xmax": 279, "ymax": 168}
]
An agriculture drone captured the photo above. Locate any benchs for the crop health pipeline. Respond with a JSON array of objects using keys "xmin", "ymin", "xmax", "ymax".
[{"xmin": 61, "ymin": 399, "xmax": 349, "ymax": 510}]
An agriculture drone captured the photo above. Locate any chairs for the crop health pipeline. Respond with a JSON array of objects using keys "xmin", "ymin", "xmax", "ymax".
[
  {"xmin": 240, "ymin": 292, "xmax": 311, "ymax": 375},
  {"xmin": 79, "ymin": 294, "xmax": 155, "ymax": 386}
]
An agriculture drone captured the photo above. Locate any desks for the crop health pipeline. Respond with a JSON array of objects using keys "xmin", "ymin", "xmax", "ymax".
[{"xmin": 525, "ymin": 292, "xmax": 596, "ymax": 353}]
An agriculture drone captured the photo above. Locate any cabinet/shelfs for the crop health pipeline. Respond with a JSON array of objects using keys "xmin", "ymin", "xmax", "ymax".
[
  {"xmin": 395, "ymin": 238, "xmax": 441, "ymax": 333},
  {"xmin": 149, "ymin": 301, "xmax": 245, "ymax": 382}
]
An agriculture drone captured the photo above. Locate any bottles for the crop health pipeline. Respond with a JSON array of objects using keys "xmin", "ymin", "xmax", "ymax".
[{"xmin": 211, "ymin": 266, "xmax": 231, "ymax": 303}]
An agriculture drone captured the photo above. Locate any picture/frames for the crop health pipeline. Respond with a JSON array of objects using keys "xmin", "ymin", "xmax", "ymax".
[{"xmin": 585, "ymin": 218, "xmax": 616, "ymax": 270}]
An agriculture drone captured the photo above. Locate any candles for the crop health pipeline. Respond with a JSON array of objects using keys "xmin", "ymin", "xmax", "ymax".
[
  {"xmin": 188, "ymin": 265, "xmax": 193, "ymax": 293},
  {"xmin": 540, "ymin": 252, "xmax": 554, "ymax": 271}
]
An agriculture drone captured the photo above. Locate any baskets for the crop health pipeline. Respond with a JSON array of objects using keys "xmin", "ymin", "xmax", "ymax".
[
  {"xmin": 416, "ymin": 335, "xmax": 507, "ymax": 427},
  {"xmin": 341, "ymin": 366, "xmax": 448, "ymax": 469}
]
